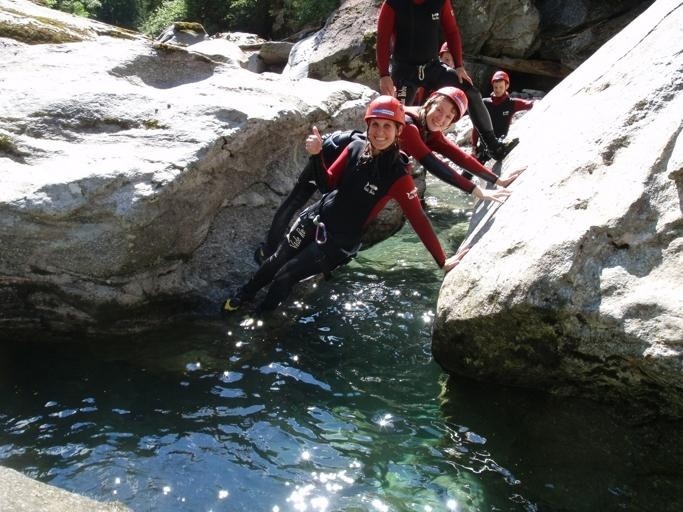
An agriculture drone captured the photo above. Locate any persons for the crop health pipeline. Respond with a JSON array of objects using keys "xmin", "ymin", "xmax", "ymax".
[
  {"xmin": 377, "ymin": 0, "xmax": 518, "ymax": 161},
  {"xmin": 255, "ymin": 87, "xmax": 527, "ymax": 265},
  {"xmin": 462, "ymin": 71, "xmax": 538, "ymax": 179},
  {"xmin": 223, "ymin": 96, "xmax": 469, "ymax": 316},
  {"xmin": 412, "ymin": 41, "xmax": 454, "ymax": 106}
]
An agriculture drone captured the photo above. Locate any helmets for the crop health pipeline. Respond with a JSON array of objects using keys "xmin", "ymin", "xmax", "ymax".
[
  {"xmin": 491, "ymin": 70, "xmax": 510, "ymax": 84},
  {"xmin": 431, "ymin": 86, "xmax": 469, "ymax": 122},
  {"xmin": 440, "ymin": 41, "xmax": 451, "ymax": 54},
  {"xmin": 365, "ymin": 95, "xmax": 406, "ymax": 128}
]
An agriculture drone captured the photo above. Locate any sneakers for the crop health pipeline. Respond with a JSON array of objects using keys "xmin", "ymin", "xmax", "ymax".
[
  {"xmin": 255, "ymin": 245, "xmax": 276, "ymax": 260},
  {"xmin": 487, "ymin": 137, "xmax": 518, "ymax": 160},
  {"xmin": 221, "ymin": 295, "xmax": 243, "ymax": 314}
]
